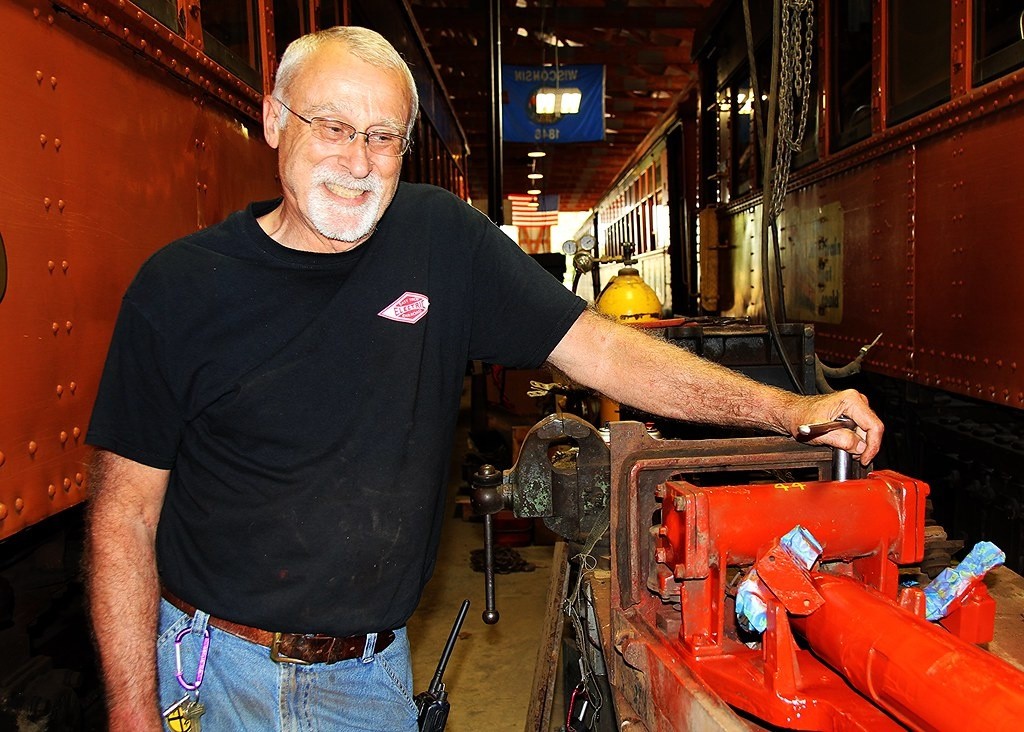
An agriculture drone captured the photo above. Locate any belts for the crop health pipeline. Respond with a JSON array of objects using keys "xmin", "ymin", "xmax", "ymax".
[{"xmin": 159, "ymin": 587, "xmax": 396, "ymax": 667}]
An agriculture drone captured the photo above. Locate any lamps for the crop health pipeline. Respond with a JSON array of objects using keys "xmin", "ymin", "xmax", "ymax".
[
  {"xmin": 527, "ymin": 141, "xmax": 546, "ymax": 158},
  {"xmin": 527, "ymin": 194, "xmax": 539, "ymax": 206},
  {"xmin": 525, "ymin": 179, "xmax": 542, "ymax": 194},
  {"xmin": 535, "ymin": 0, "xmax": 582, "ymax": 115},
  {"xmin": 526, "ymin": 158, "xmax": 543, "ymax": 179}
]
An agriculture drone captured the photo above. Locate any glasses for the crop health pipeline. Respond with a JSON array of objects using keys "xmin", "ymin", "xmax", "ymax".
[{"xmin": 273, "ymin": 96, "xmax": 415, "ymax": 157}]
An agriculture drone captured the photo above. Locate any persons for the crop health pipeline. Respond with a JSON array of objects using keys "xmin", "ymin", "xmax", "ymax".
[{"xmin": 84, "ymin": 26, "xmax": 884, "ymax": 732}]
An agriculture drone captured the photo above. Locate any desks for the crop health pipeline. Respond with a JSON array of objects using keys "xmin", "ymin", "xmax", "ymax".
[{"xmin": 403, "ymin": 475, "xmax": 1024, "ymax": 732}]
